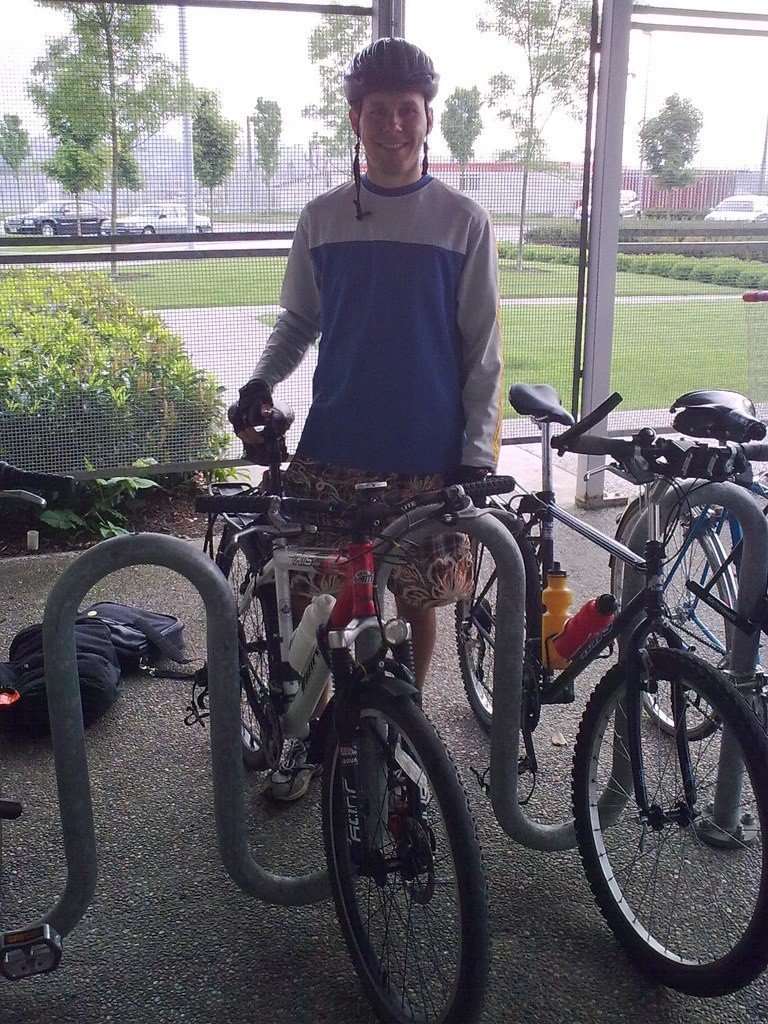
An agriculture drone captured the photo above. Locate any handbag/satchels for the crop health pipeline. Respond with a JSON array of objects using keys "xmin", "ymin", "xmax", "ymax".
[{"xmin": 75, "ymin": 602, "xmax": 185, "ymax": 670}]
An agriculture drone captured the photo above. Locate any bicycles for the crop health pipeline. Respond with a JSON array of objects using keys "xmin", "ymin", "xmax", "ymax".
[
  {"xmin": 453, "ymin": 382, "xmax": 766, "ymax": 999},
  {"xmin": 197, "ymin": 403, "xmax": 515, "ymax": 1021},
  {"xmin": 605, "ymin": 388, "xmax": 768, "ymax": 742}
]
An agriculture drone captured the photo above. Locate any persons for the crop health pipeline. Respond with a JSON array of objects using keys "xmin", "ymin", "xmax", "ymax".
[{"xmin": 229, "ymin": 38, "xmax": 505, "ymax": 818}]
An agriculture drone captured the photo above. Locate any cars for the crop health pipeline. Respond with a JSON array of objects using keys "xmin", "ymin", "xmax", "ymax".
[
  {"xmin": 3, "ymin": 199, "xmax": 110, "ymax": 235},
  {"xmin": 573, "ymin": 190, "xmax": 644, "ymax": 224},
  {"xmin": 100, "ymin": 203, "xmax": 213, "ymax": 235},
  {"xmin": 704, "ymin": 195, "xmax": 768, "ymax": 226}
]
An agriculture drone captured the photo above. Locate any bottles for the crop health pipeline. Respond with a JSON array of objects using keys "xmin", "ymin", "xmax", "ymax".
[
  {"xmin": 553, "ymin": 595, "xmax": 617, "ymax": 659},
  {"xmin": 541, "ymin": 561, "xmax": 573, "ymax": 668},
  {"xmin": 288, "ymin": 594, "xmax": 336, "ymax": 676}
]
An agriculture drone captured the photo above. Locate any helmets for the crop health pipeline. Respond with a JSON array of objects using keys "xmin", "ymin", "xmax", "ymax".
[{"xmin": 344, "ymin": 37, "xmax": 440, "ymax": 105}]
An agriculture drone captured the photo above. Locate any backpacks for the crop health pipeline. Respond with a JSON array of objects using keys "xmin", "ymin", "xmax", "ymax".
[{"xmin": 0, "ymin": 618, "xmax": 121, "ymax": 736}]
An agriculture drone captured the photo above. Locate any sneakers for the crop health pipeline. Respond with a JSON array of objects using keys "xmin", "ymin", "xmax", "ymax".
[
  {"xmin": 268, "ymin": 738, "xmax": 324, "ymax": 801},
  {"xmin": 389, "ymin": 769, "xmax": 430, "ymax": 810}
]
